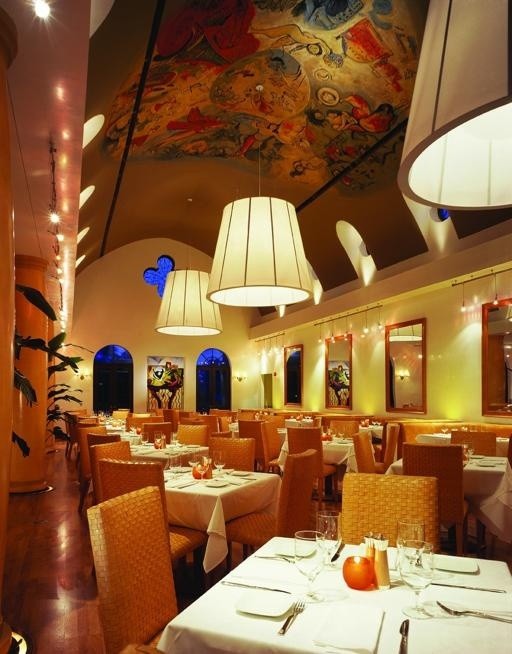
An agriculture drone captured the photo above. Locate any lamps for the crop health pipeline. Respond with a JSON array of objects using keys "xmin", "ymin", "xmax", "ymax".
[
  {"xmin": 396, "ymin": 0, "xmax": 512, "ymax": 213},
  {"xmin": 399, "ymin": 370, "xmax": 409, "ymax": 380},
  {"xmin": 205, "ymin": 86, "xmax": 311, "ymax": 309},
  {"xmin": 154, "ymin": 269, "xmax": 223, "ymax": 336}
]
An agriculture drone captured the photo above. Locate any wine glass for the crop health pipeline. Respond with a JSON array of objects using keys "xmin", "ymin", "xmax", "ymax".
[
  {"xmin": 97, "ymin": 412, "xmax": 178, "ymax": 452},
  {"xmin": 440, "ymin": 423, "xmax": 469, "ymax": 435},
  {"xmin": 164, "ymin": 446, "xmax": 257, "ymax": 489},
  {"xmin": 293, "ymin": 509, "xmax": 435, "ymax": 622},
  {"xmin": 371, "ymin": 416, "xmax": 382, "ymax": 429}
]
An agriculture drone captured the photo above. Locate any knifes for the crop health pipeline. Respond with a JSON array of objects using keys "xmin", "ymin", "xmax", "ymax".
[
  {"xmin": 395, "ymin": 619, "xmax": 411, "ymax": 654},
  {"xmin": 430, "ymin": 582, "xmax": 506, "ymax": 595},
  {"xmin": 220, "ymin": 580, "xmax": 292, "ymax": 595}
]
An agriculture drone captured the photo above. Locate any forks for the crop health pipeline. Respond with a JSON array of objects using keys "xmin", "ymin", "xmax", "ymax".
[
  {"xmin": 277, "ymin": 599, "xmax": 305, "ymax": 635},
  {"xmin": 435, "ymin": 600, "xmax": 511, "ymax": 623}
]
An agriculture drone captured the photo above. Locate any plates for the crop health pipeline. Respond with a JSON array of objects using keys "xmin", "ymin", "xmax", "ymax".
[
  {"xmin": 469, "ymin": 454, "xmax": 507, "ymax": 468},
  {"xmin": 431, "ymin": 556, "xmax": 480, "ymax": 574},
  {"xmin": 232, "ymin": 592, "xmax": 294, "ymax": 618}
]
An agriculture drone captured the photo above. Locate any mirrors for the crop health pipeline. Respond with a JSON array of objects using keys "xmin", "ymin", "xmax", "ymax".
[
  {"xmin": 284, "ymin": 345, "xmax": 303, "ymax": 406},
  {"xmin": 385, "ymin": 317, "xmax": 427, "ymax": 414},
  {"xmin": 324, "ymin": 334, "xmax": 352, "ymax": 410},
  {"xmin": 481, "ymin": 296, "xmax": 512, "ymax": 417}
]
